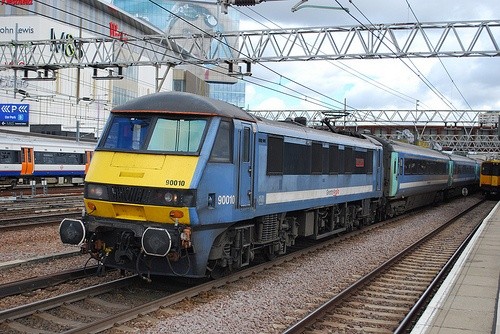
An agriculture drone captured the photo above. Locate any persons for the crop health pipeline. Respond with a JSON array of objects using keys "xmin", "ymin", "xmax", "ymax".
[{"xmin": 163, "ymin": 2, "xmax": 240, "ymax": 86}]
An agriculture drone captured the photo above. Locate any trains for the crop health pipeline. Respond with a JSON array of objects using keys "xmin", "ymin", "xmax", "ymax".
[
  {"xmin": 0, "ymin": 129, "xmax": 102, "ymax": 189},
  {"xmin": 58, "ymin": 90, "xmax": 482, "ymax": 280},
  {"xmin": 480, "ymin": 159, "xmax": 500, "ymax": 200}
]
